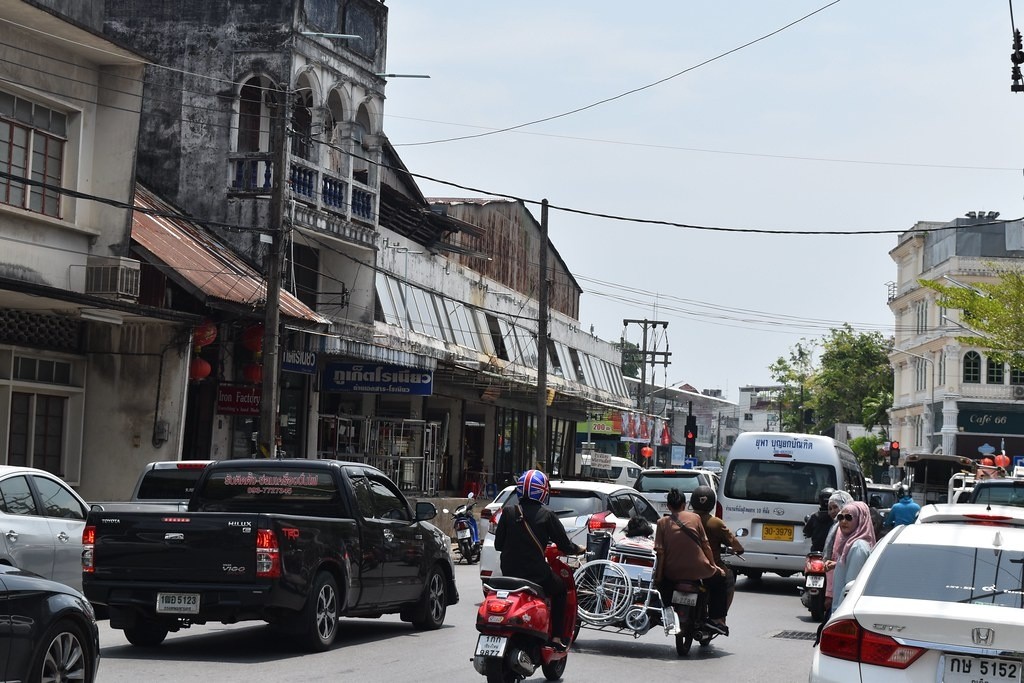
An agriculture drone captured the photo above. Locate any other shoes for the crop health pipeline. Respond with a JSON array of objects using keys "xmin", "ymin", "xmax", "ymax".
[
  {"xmin": 706, "ymin": 620, "xmax": 729, "ymax": 636},
  {"xmin": 552, "ymin": 641, "xmax": 567, "ymax": 651}
]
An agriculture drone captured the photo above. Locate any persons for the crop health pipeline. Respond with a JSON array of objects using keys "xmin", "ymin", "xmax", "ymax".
[
  {"xmin": 803, "ymin": 488, "xmax": 876, "ymax": 616},
  {"xmin": 884, "ymin": 487, "xmax": 920, "ymax": 526},
  {"xmin": 495, "ymin": 470, "xmax": 587, "ymax": 651},
  {"xmin": 654, "ymin": 486, "xmax": 744, "ymax": 636}
]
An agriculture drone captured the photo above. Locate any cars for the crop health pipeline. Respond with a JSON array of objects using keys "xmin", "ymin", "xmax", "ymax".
[
  {"xmin": 0, "ymin": 465, "xmax": 104, "ymax": 604},
  {"xmin": 810, "ymin": 523, "xmax": 1024, "ymax": 683},
  {"xmin": 0, "ymin": 563, "xmax": 101, "ymax": 682},
  {"xmin": 480, "ymin": 480, "xmax": 672, "ymax": 598},
  {"xmin": 701, "ymin": 461, "xmax": 723, "ymax": 476},
  {"xmin": 915, "ymin": 504, "xmax": 1024, "ymax": 527}
]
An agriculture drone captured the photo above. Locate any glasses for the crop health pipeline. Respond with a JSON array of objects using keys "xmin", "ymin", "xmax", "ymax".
[{"xmin": 837, "ymin": 513, "xmax": 853, "ymax": 521}]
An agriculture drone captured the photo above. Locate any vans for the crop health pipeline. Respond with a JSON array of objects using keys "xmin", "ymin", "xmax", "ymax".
[
  {"xmin": 579, "ymin": 454, "xmax": 643, "ymax": 489},
  {"xmin": 714, "ymin": 431, "xmax": 882, "ymax": 580}
]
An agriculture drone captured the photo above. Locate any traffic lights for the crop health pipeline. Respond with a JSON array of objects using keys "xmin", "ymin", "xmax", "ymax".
[
  {"xmin": 890, "ymin": 442, "xmax": 899, "ymax": 466},
  {"xmin": 684, "ymin": 416, "xmax": 699, "ymax": 457}
]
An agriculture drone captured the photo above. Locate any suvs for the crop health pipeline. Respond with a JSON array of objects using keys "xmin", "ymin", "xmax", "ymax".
[
  {"xmin": 865, "ymin": 483, "xmax": 898, "ymax": 517},
  {"xmin": 634, "ymin": 467, "xmax": 720, "ymax": 520},
  {"xmin": 969, "ymin": 480, "xmax": 1024, "ymax": 507}
]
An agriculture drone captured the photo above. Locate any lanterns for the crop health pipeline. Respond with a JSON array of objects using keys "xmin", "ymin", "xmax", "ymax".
[
  {"xmin": 190, "ymin": 356, "xmax": 211, "ymax": 381},
  {"xmin": 243, "ymin": 361, "xmax": 263, "ymax": 384},
  {"xmin": 194, "ymin": 320, "xmax": 217, "ymax": 352},
  {"xmin": 243, "ymin": 324, "xmax": 264, "ymax": 356},
  {"xmin": 983, "ymin": 458, "xmax": 993, "ymax": 465},
  {"xmin": 995, "ymin": 455, "xmax": 1010, "ymax": 467},
  {"xmin": 642, "ymin": 447, "xmax": 653, "ymax": 457}
]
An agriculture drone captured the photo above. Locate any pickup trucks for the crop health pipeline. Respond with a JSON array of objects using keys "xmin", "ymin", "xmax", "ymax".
[
  {"xmin": 948, "ymin": 473, "xmax": 1024, "ymax": 504},
  {"xmin": 86, "ymin": 461, "xmax": 214, "ymax": 512},
  {"xmin": 82, "ymin": 455, "xmax": 461, "ymax": 650}
]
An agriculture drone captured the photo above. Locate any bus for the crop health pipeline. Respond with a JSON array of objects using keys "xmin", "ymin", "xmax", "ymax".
[{"xmin": 903, "ymin": 455, "xmax": 978, "ymax": 504}]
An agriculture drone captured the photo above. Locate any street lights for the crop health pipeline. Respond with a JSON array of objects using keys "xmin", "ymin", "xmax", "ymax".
[{"xmin": 879, "ymin": 345, "xmax": 934, "ymax": 453}]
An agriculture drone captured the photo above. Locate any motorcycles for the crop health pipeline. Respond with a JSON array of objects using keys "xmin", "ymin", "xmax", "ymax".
[
  {"xmin": 442, "ymin": 491, "xmax": 484, "ymax": 565},
  {"xmin": 573, "ymin": 524, "xmax": 748, "ymax": 655}
]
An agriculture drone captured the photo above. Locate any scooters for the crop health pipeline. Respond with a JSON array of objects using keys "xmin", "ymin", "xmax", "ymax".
[{"xmin": 469, "ymin": 517, "xmax": 596, "ymax": 683}]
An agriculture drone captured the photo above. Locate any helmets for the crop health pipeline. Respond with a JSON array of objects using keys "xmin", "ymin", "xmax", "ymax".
[
  {"xmin": 819, "ymin": 487, "xmax": 835, "ymax": 509},
  {"xmin": 690, "ymin": 485, "xmax": 716, "ymax": 513},
  {"xmin": 516, "ymin": 470, "xmax": 550, "ymax": 506},
  {"xmin": 897, "ymin": 484, "xmax": 912, "ymax": 498}
]
